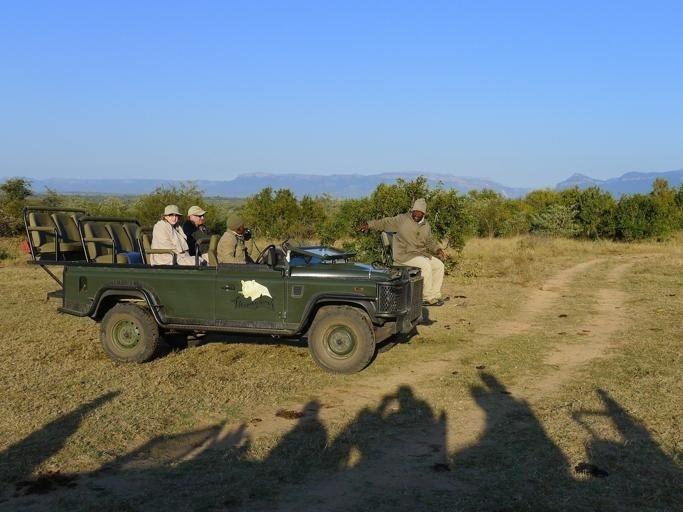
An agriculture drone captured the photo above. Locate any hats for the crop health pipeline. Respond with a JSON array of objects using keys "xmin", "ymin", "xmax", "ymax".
[
  {"xmin": 410, "ymin": 198, "xmax": 427, "ymax": 214},
  {"xmin": 163, "ymin": 204, "xmax": 183, "ymax": 215},
  {"xmin": 187, "ymin": 206, "xmax": 208, "ymax": 217},
  {"xmin": 227, "ymin": 214, "xmax": 244, "ymax": 230}
]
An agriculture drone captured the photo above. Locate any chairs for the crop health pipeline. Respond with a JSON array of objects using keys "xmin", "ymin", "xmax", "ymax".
[
  {"xmin": 380, "ymin": 231, "xmax": 394, "ymax": 265},
  {"xmin": 83, "ymin": 221, "xmax": 153, "ymax": 267},
  {"xmin": 209, "ymin": 233, "xmax": 221, "ymax": 265},
  {"xmin": 30, "ymin": 212, "xmax": 84, "ymax": 258}
]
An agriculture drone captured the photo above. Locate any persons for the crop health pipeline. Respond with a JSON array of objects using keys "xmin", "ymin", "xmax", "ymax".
[
  {"xmin": 150, "ymin": 205, "xmax": 210, "ymax": 266},
  {"xmin": 356, "ymin": 198, "xmax": 444, "ymax": 306},
  {"xmin": 217, "ymin": 216, "xmax": 245, "ymax": 264}
]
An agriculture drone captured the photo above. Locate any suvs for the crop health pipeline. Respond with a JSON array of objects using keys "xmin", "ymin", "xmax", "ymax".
[{"xmin": 22, "ymin": 206, "xmax": 424, "ymax": 375}]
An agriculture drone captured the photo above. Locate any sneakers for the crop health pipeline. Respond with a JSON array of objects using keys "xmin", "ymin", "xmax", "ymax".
[{"xmin": 422, "ymin": 299, "xmax": 443, "ymax": 306}]
